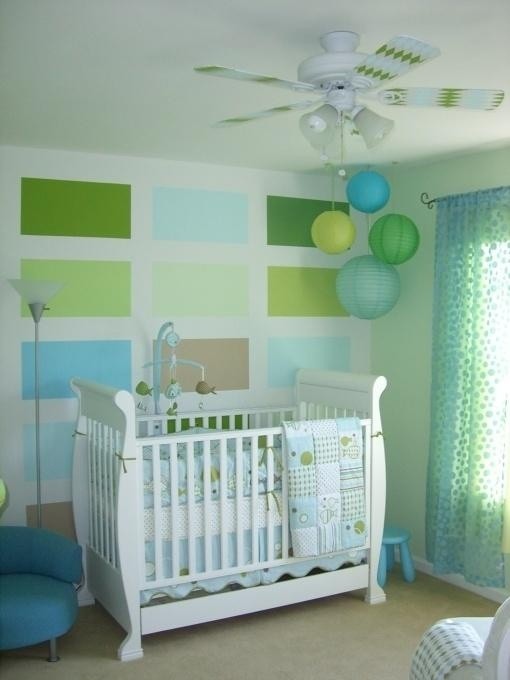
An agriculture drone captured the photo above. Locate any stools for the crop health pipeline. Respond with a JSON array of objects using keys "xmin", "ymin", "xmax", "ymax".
[{"xmin": 377, "ymin": 524, "xmax": 415, "ymax": 587}]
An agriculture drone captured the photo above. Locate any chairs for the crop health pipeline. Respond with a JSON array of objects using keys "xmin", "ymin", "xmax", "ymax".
[
  {"xmin": 408, "ymin": 596, "xmax": 510, "ymax": 680},
  {"xmin": 0, "ymin": 524, "xmax": 83, "ymax": 662}
]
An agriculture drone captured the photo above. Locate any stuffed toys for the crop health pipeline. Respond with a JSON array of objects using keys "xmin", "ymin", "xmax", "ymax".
[{"xmin": 134, "ymin": 321, "xmax": 217, "ymax": 417}]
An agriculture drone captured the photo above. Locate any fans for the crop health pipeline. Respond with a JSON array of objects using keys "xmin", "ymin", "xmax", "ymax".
[{"xmin": 191, "ymin": 30, "xmax": 505, "ymax": 128}]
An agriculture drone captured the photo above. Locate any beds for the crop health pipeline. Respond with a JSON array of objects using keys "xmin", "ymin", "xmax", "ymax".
[{"xmin": 67, "ymin": 368, "xmax": 388, "ymax": 663}]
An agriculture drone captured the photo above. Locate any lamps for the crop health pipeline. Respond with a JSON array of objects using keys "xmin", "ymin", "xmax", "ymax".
[
  {"xmin": 368, "ymin": 213, "xmax": 420, "ymax": 265},
  {"xmin": 301, "ymin": 105, "xmax": 395, "ymax": 158},
  {"xmin": 336, "ymin": 254, "xmax": 402, "ymax": 320},
  {"xmin": 346, "ymin": 170, "xmax": 390, "ymax": 213},
  {"xmin": 310, "ymin": 174, "xmax": 356, "ymax": 254},
  {"xmin": 8, "ymin": 278, "xmax": 65, "ymax": 528}
]
